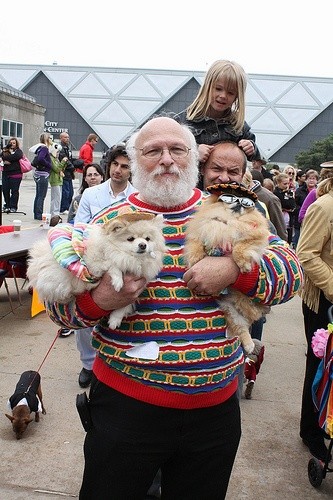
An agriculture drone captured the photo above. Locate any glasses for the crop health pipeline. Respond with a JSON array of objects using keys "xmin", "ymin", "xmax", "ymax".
[
  {"xmin": 289, "ymin": 172, "xmax": 295, "ymax": 175},
  {"xmin": 87, "ymin": 173, "xmax": 100, "ymax": 177},
  {"xmin": 217, "ymin": 193, "xmax": 255, "ymax": 210},
  {"xmin": 139, "ymin": 142, "xmax": 191, "ymax": 159}
]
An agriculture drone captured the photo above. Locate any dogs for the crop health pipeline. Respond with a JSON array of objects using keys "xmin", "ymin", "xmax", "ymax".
[
  {"xmin": 243, "ymin": 339, "xmax": 265, "ymax": 399},
  {"xmin": 2, "ymin": 143, "xmax": 12, "ymax": 160},
  {"xmin": 5, "ymin": 370, "xmax": 46, "ymax": 440},
  {"xmin": 184, "ymin": 194, "xmax": 272, "ymax": 352},
  {"xmin": 26, "ymin": 211, "xmax": 167, "ymax": 330}
]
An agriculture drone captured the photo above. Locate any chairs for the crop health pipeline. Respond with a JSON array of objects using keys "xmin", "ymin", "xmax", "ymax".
[{"xmin": 0, "ymin": 226, "xmax": 29, "ymax": 312}]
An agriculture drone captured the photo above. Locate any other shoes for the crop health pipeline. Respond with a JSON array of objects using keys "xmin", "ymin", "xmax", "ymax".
[
  {"xmin": 3, "ymin": 207, "xmax": 10, "ymax": 211},
  {"xmin": 10, "ymin": 208, "xmax": 16, "ymax": 212},
  {"xmin": 79, "ymin": 368, "xmax": 93, "ymax": 387},
  {"xmin": 302, "ymin": 437, "xmax": 332, "ymax": 462},
  {"xmin": 61, "ymin": 328, "xmax": 72, "ymax": 336},
  {"xmin": 60, "ymin": 210, "xmax": 69, "ymax": 215}
]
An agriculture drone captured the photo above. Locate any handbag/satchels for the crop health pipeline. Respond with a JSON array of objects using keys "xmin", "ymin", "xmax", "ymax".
[{"xmin": 19, "ymin": 157, "xmax": 32, "ymax": 173}]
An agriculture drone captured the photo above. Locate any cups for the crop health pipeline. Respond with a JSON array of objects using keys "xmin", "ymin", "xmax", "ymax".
[
  {"xmin": 42, "ymin": 213, "xmax": 51, "ymax": 229},
  {"xmin": 13, "ymin": 220, "xmax": 21, "ymax": 234}
]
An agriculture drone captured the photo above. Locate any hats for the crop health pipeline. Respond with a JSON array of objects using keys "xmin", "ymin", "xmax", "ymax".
[{"xmin": 320, "ymin": 161, "xmax": 333, "ymax": 168}]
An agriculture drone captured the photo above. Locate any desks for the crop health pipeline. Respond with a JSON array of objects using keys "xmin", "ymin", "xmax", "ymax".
[{"xmin": 0, "ymin": 228, "xmax": 57, "ymax": 313}]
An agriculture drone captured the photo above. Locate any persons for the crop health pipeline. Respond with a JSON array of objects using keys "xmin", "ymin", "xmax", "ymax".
[
  {"xmin": 72, "ymin": 145, "xmax": 139, "ymax": 387},
  {"xmin": 190, "ymin": 138, "xmax": 270, "ymax": 343},
  {"xmin": 170, "ymin": 59, "xmax": 261, "ymax": 167},
  {"xmin": 56, "ymin": 163, "xmax": 105, "ymax": 337},
  {"xmin": 44, "ymin": 117, "xmax": 305, "ymax": 500},
  {"xmin": 252, "ymin": 157, "xmax": 333, "ymax": 468},
  {"xmin": 0, "ymin": 126, "xmax": 98, "ymax": 220}
]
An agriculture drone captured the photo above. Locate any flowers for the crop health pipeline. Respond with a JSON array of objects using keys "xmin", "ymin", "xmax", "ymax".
[{"xmin": 311, "ymin": 323, "xmax": 333, "ymax": 359}]
